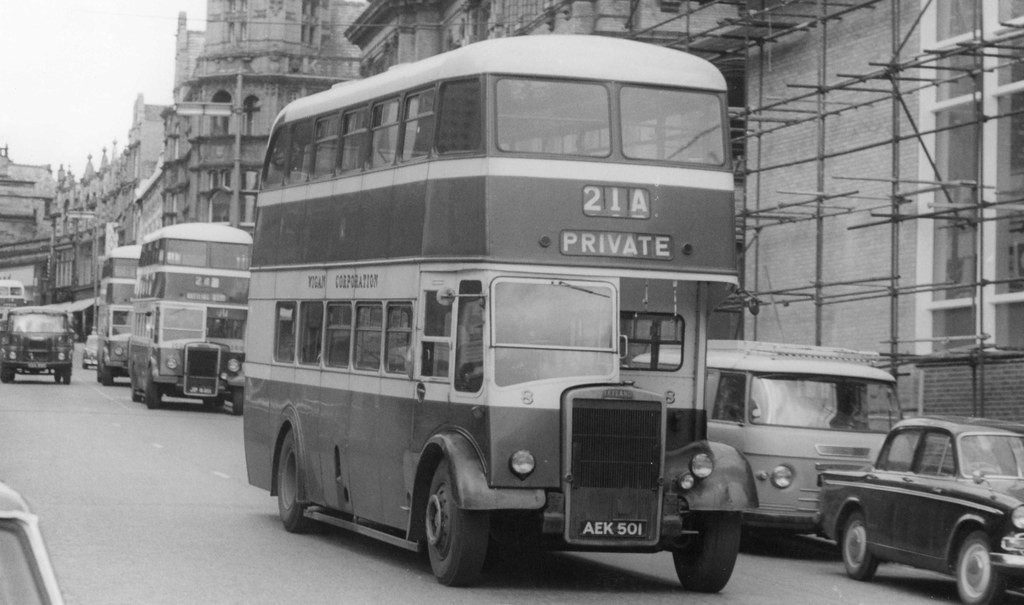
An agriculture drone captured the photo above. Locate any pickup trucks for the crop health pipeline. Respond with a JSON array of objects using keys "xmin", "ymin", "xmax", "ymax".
[{"xmin": 816, "ymin": 415, "xmax": 1024, "ymax": 603}]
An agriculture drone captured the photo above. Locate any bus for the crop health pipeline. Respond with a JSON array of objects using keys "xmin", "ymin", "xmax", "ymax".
[
  {"xmin": 127, "ymin": 223, "xmax": 253, "ymax": 415},
  {"xmin": 0, "ymin": 280, "xmax": 27, "ymax": 332},
  {"xmin": 244, "ymin": 34, "xmax": 742, "ymax": 592},
  {"xmin": 98, "ymin": 245, "xmax": 181, "ymax": 386}
]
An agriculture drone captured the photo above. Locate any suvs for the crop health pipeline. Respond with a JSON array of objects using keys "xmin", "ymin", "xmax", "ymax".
[{"xmin": 1, "ymin": 308, "xmax": 73, "ymax": 383}]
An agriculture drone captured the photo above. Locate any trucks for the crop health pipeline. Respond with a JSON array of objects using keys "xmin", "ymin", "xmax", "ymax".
[{"xmin": 633, "ymin": 337, "xmax": 902, "ymax": 539}]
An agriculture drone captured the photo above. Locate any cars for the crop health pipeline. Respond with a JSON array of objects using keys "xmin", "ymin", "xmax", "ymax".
[{"xmin": 82, "ymin": 334, "xmax": 100, "ymax": 369}]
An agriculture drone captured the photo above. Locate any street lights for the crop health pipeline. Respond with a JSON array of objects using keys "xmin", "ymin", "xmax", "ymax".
[
  {"xmin": 67, "ymin": 211, "xmax": 101, "ymax": 336},
  {"xmin": 174, "ymin": 72, "xmax": 244, "ymax": 229}
]
